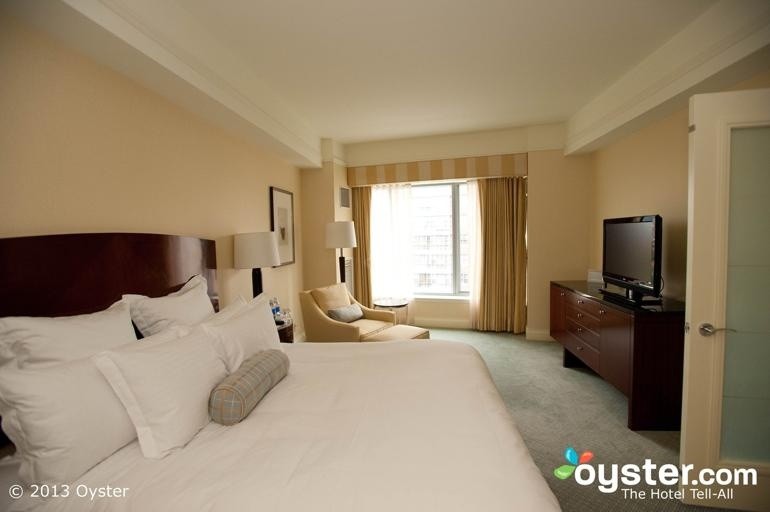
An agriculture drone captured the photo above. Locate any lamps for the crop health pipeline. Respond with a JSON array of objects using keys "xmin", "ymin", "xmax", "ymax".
[
  {"xmin": 233, "ymin": 230, "xmax": 282, "ymax": 299},
  {"xmin": 323, "ymin": 219, "xmax": 359, "ymax": 284}
]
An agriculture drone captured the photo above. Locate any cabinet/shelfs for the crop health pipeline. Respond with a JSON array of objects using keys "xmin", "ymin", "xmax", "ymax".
[{"xmin": 547, "ymin": 277, "xmax": 686, "ymax": 432}]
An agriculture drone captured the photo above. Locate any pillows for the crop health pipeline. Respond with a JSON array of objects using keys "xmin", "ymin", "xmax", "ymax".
[
  {"xmin": 120, "ymin": 273, "xmax": 216, "ymax": 338},
  {"xmin": 309, "ymin": 282, "xmax": 351, "ymax": 316},
  {"xmin": 202, "ymin": 293, "xmax": 246, "ymax": 331},
  {"xmin": 1, "ymin": 298, "xmax": 145, "ymax": 497},
  {"xmin": 208, "ymin": 347, "xmax": 292, "ymax": 428},
  {"xmin": 200, "ymin": 287, "xmax": 279, "ymax": 374},
  {"xmin": 91, "ymin": 316, "xmax": 229, "ymax": 461},
  {"xmin": 327, "ymin": 302, "xmax": 364, "ymax": 324}
]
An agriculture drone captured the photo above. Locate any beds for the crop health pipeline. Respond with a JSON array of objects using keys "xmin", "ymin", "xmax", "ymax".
[{"xmin": 0, "ymin": 231, "xmax": 566, "ymax": 511}]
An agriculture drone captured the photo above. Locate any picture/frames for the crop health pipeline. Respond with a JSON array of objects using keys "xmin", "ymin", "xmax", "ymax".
[{"xmin": 268, "ymin": 184, "xmax": 297, "ymax": 268}]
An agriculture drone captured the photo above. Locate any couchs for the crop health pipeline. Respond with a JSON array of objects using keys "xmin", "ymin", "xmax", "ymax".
[{"xmin": 304, "ymin": 282, "xmax": 432, "ymax": 341}]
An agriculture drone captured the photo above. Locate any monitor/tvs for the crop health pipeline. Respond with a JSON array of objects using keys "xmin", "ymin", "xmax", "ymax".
[{"xmin": 598, "ymin": 214, "xmax": 663, "ymax": 306}]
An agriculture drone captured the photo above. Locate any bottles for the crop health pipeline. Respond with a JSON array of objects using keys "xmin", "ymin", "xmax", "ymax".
[
  {"xmin": 269, "ymin": 300, "xmax": 278, "ymax": 319},
  {"xmin": 274, "ymin": 296, "xmax": 281, "ymax": 314}
]
